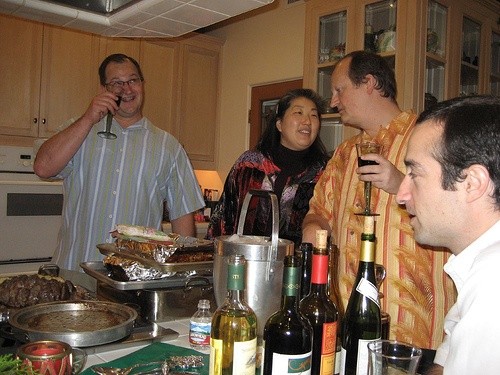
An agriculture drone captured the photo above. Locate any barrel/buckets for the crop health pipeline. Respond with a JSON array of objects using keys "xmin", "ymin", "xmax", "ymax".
[{"xmin": 213, "ymin": 189, "xmax": 294, "ymax": 335}]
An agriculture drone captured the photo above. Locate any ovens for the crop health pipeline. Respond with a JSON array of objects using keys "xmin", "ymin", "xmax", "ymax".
[{"xmin": 0, "ymin": 145, "xmax": 65, "ymax": 260}]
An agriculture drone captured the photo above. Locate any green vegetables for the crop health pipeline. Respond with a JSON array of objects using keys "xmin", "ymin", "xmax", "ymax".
[{"xmin": 0, "ymin": 353, "xmax": 44, "ymax": 375}]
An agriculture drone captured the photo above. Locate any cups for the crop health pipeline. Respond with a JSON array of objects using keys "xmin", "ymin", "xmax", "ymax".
[
  {"xmin": 380, "ymin": 311, "xmax": 390, "ymax": 375},
  {"xmin": 16, "ymin": 340, "xmax": 88, "ymax": 375},
  {"xmin": 366, "ymin": 340, "xmax": 423, "ymax": 375}
]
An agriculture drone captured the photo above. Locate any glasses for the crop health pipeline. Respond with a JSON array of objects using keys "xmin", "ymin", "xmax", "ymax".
[{"xmin": 105, "ymin": 76, "xmax": 144, "ymax": 90}]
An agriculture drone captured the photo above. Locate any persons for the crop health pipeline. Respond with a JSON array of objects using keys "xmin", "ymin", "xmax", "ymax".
[
  {"xmin": 395, "ymin": 96, "xmax": 500, "ymax": 375},
  {"xmin": 300, "ymin": 49, "xmax": 458, "ymax": 349},
  {"xmin": 32, "ymin": 53, "xmax": 205, "ymax": 273},
  {"xmin": 206, "ymin": 89, "xmax": 331, "ymax": 241}
]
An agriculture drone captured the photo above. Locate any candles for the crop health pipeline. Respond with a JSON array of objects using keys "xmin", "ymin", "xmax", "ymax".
[{"xmin": 22, "ymin": 348, "xmax": 71, "ymax": 375}]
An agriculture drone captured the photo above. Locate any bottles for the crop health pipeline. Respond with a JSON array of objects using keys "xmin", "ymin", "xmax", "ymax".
[
  {"xmin": 295, "ymin": 242, "xmax": 314, "ymax": 301},
  {"xmin": 364, "ymin": 25, "xmax": 375, "ymax": 54},
  {"xmin": 189, "ymin": 299, "xmax": 213, "ymax": 350},
  {"xmin": 208, "ymin": 254, "xmax": 257, "ymax": 375},
  {"xmin": 341, "ymin": 216, "xmax": 383, "ymax": 375},
  {"xmin": 326, "ymin": 244, "xmax": 343, "ymax": 375},
  {"xmin": 261, "ymin": 255, "xmax": 313, "ymax": 375},
  {"xmin": 299, "ymin": 230, "xmax": 339, "ymax": 375}
]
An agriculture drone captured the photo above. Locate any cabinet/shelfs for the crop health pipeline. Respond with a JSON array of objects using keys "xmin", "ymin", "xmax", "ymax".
[
  {"xmin": 302, "ymin": 0, "xmax": 500, "ymax": 159},
  {"xmin": 0, "ymin": 14, "xmax": 100, "ymax": 147},
  {"xmin": 100, "ymin": 32, "xmax": 224, "ymax": 170}
]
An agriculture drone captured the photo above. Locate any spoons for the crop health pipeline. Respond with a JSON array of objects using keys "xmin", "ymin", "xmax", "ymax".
[{"xmin": 91, "ymin": 361, "xmax": 163, "ymax": 375}]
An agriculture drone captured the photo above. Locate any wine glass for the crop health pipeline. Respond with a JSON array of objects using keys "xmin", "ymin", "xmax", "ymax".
[
  {"xmin": 354, "ymin": 143, "xmax": 381, "ymax": 216},
  {"xmin": 97, "ymin": 85, "xmax": 124, "ymax": 140}
]
